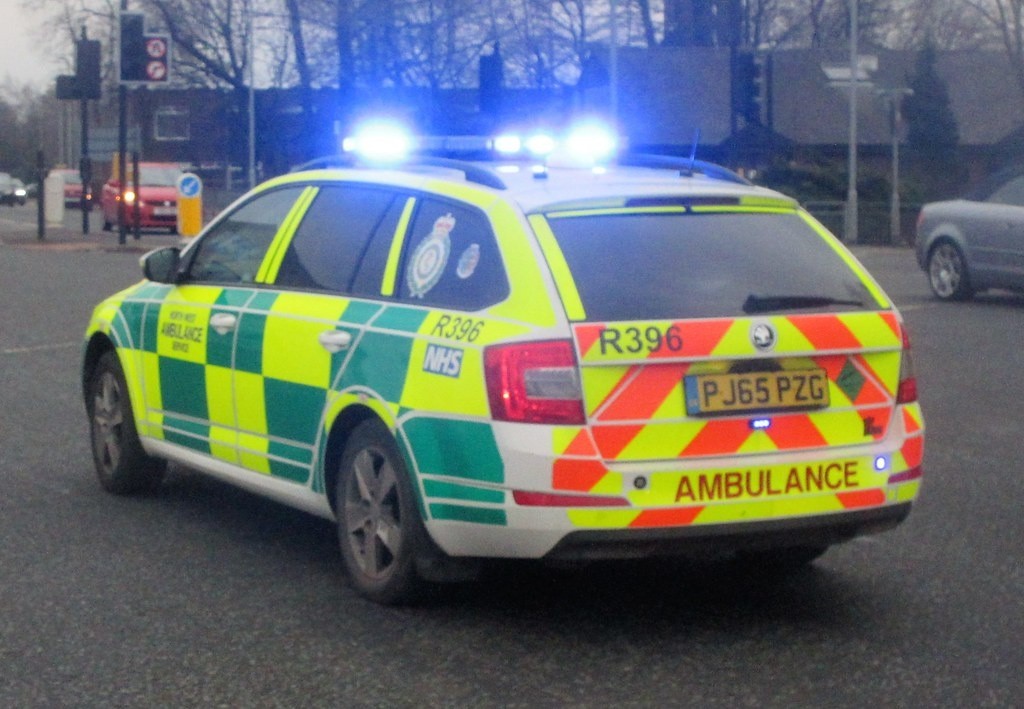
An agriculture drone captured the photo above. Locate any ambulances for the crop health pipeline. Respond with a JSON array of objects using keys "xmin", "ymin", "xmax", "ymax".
[{"xmin": 81, "ymin": 128, "xmax": 926, "ymax": 608}]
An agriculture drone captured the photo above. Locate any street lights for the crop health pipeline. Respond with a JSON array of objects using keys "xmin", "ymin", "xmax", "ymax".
[{"xmin": 877, "ymin": 89, "xmax": 915, "ymax": 242}]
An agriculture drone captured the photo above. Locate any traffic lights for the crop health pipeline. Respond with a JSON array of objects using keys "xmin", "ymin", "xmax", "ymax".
[
  {"xmin": 119, "ymin": 13, "xmax": 146, "ymax": 80},
  {"xmin": 734, "ymin": 54, "xmax": 758, "ymax": 118}
]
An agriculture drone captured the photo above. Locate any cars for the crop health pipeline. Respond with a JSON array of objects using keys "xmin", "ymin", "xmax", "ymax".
[
  {"xmin": 0, "ymin": 174, "xmax": 26, "ymax": 205},
  {"xmin": 913, "ymin": 164, "xmax": 1024, "ymax": 298},
  {"xmin": 49, "ymin": 168, "xmax": 91, "ymax": 206},
  {"xmin": 101, "ymin": 162, "xmax": 185, "ymax": 237}
]
